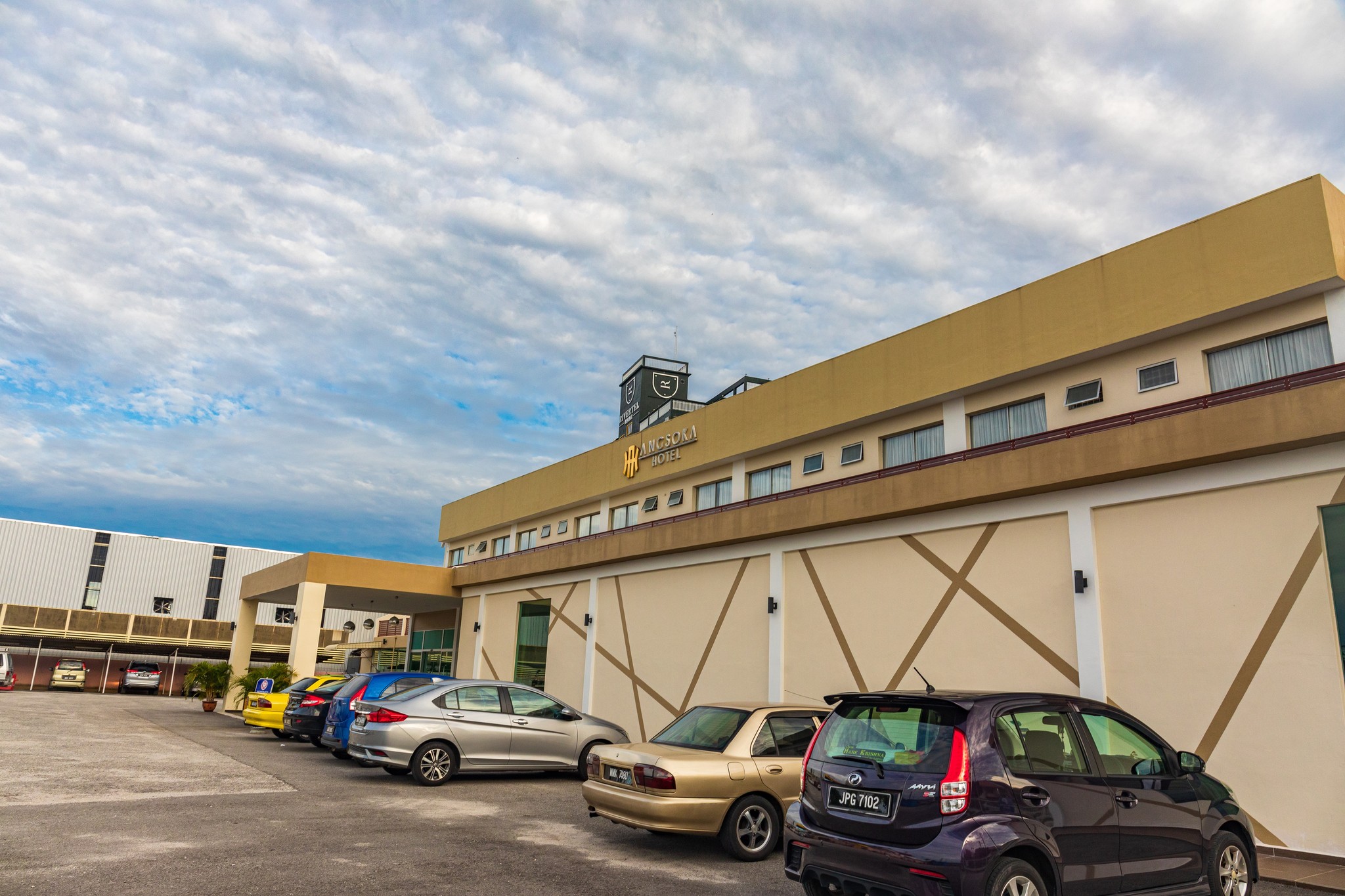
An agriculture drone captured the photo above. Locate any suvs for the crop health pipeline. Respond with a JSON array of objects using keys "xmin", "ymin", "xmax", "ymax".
[
  {"xmin": 780, "ymin": 686, "xmax": 1260, "ymax": 896},
  {"xmin": 117, "ymin": 660, "xmax": 162, "ymax": 696}
]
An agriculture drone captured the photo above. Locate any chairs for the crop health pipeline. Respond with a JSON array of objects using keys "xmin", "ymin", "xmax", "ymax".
[
  {"xmin": 1023, "ymin": 728, "xmax": 1063, "ymax": 771},
  {"xmin": 485, "ymin": 695, "xmax": 501, "ymax": 711},
  {"xmin": 996, "ymin": 727, "xmax": 1015, "ymax": 760},
  {"xmin": 782, "ymin": 727, "xmax": 813, "ymax": 744},
  {"xmin": 473, "ymin": 695, "xmax": 483, "ymax": 709}
]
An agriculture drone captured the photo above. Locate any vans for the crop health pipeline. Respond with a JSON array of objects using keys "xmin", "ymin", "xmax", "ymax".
[
  {"xmin": 48, "ymin": 659, "xmax": 90, "ymax": 692},
  {"xmin": 0, "ymin": 651, "xmax": 14, "ymax": 687}
]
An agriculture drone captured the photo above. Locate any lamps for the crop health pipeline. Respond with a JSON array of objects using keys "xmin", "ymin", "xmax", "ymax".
[
  {"xmin": 383, "ymin": 638, "xmax": 387, "ymax": 645},
  {"xmin": 343, "ymin": 603, "xmax": 355, "ymax": 633},
  {"xmin": 768, "ymin": 597, "xmax": 777, "ymax": 614},
  {"xmin": 363, "ymin": 600, "xmax": 375, "ymax": 630},
  {"xmin": 389, "ymin": 596, "xmax": 399, "ymax": 627},
  {"xmin": 584, "ymin": 613, "xmax": 592, "ymax": 627},
  {"xmin": 473, "ymin": 622, "xmax": 480, "ymax": 632},
  {"xmin": 290, "ymin": 612, "xmax": 298, "ymax": 624},
  {"xmin": 230, "ymin": 621, "xmax": 237, "ymax": 632},
  {"xmin": 1074, "ymin": 570, "xmax": 1088, "ymax": 595}
]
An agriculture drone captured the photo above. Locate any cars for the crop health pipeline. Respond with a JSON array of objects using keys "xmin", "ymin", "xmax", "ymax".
[
  {"xmin": 581, "ymin": 700, "xmax": 911, "ymax": 862},
  {"xmin": 243, "ymin": 675, "xmax": 347, "ymax": 739},
  {"xmin": 319, "ymin": 668, "xmax": 497, "ymax": 768},
  {"xmin": 181, "ymin": 669, "xmax": 219, "ymax": 698},
  {"xmin": 283, "ymin": 672, "xmax": 391, "ymax": 748},
  {"xmin": 346, "ymin": 677, "xmax": 633, "ymax": 786}
]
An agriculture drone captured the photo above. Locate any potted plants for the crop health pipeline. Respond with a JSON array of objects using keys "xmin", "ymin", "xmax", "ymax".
[{"xmin": 183, "ymin": 658, "xmax": 235, "ymax": 711}]
